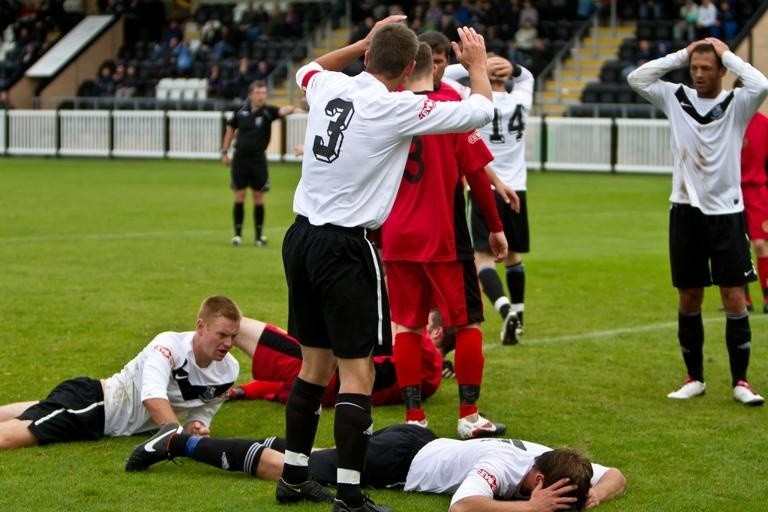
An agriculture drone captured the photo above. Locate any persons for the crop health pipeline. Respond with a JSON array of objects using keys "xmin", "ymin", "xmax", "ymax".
[
  {"xmin": 1, "ymin": 1, "xmax": 92, "ymax": 116},
  {"xmin": 343, "ymin": 1, "xmax": 603, "ymax": 86},
  {"xmin": 419, "ymin": 29, "xmax": 520, "ymax": 379},
  {"xmin": 383, "ymin": 42, "xmax": 510, "ymax": 438},
  {"xmin": 438, "ymin": 52, "xmax": 535, "ymax": 347},
  {"xmin": 0, "ymin": 294, "xmax": 241, "ymax": 452},
  {"xmin": 218, "ymin": 81, "xmax": 306, "ymax": 247},
  {"xmin": 229, "ymin": 308, "xmax": 454, "ymax": 408},
  {"xmin": 728, "ymin": 74, "xmax": 768, "ymax": 315},
  {"xmin": 274, "ymin": 14, "xmax": 497, "ymax": 511},
  {"xmin": 623, "ymin": 1, "xmax": 737, "ymax": 85},
  {"xmin": 627, "ymin": 38, "xmax": 768, "ymax": 404},
  {"xmin": 82, "ymin": 0, "xmax": 348, "ymax": 113},
  {"xmin": 123, "ymin": 419, "xmax": 628, "ymax": 510}
]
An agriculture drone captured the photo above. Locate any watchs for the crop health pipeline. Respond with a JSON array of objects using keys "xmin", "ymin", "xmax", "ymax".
[{"xmin": 220, "ymin": 149, "xmax": 229, "ymax": 155}]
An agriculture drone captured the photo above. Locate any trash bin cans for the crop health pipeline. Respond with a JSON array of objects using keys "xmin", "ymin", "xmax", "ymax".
[{"xmin": 220, "ymin": 149, "xmax": 229, "ymax": 155}]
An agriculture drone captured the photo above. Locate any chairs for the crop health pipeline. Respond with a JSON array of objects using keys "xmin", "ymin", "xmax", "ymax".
[
  {"xmin": 125, "ymin": 423, "xmax": 187, "ymax": 472},
  {"xmin": 499, "ymin": 309, "xmax": 523, "ymax": 344},
  {"xmin": 732, "ymin": 380, "xmax": 764, "ymax": 404},
  {"xmin": 275, "ymin": 477, "xmax": 395, "ymax": 511},
  {"xmin": 666, "ymin": 376, "xmax": 705, "ymax": 399},
  {"xmin": 457, "ymin": 412, "xmax": 505, "ymax": 440},
  {"xmin": 0, "ymin": 0, "xmax": 763, "ymax": 118}
]
